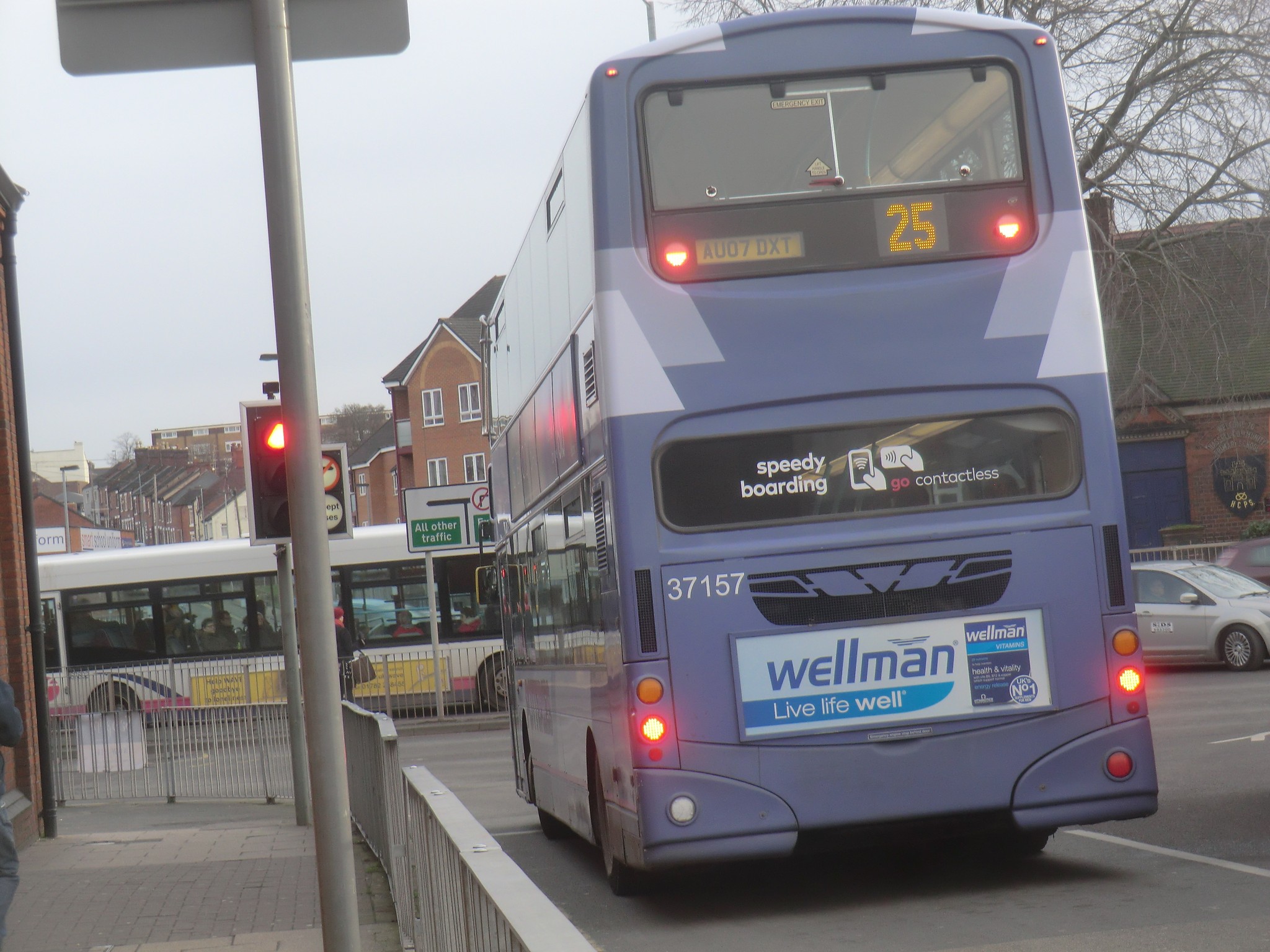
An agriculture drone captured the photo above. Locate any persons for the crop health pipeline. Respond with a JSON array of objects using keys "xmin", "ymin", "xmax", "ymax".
[
  {"xmin": 333, "ymin": 607, "xmax": 366, "ymax": 703},
  {"xmin": 69, "ymin": 598, "xmax": 280, "ymax": 657},
  {"xmin": 393, "ymin": 611, "xmax": 423, "ymax": 637},
  {"xmin": 459, "ymin": 607, "xmax": 481, "ymax": 633}
]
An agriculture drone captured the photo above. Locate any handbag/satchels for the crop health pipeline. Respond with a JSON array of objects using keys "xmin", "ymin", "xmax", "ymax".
[{"xmin": 347, "ymin": 647, "xmax": 376, "ymax": 684}]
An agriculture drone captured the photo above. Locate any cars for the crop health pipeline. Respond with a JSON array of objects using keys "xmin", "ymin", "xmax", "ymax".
[
  {"xmin": 1212, "ymin": 537, "xmax": 1270, "ymax": 592},
  {"xmin": 1126, "ymin": 559, "xmax": 1270, "ymax": 672}
]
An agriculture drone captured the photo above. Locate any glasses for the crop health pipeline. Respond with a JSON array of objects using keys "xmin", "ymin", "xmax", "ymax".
[{"xmin": 208, "ymin": 625, "xmax": 215, "ymax": 628}]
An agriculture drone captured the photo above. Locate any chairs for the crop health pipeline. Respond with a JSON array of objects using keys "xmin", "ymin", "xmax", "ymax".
[{"xmin": 102, "ymin": 617, "xmax": 460, "ymax": 658}]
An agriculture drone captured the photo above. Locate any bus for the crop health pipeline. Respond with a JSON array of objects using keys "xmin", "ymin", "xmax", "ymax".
[
  {"xmin": 35, "ymin": 515, "xmax": 508, "ymax": 735},
  {"xmin": 471, "ymin": 4, "xmax": 1164, "ymax": 901}
]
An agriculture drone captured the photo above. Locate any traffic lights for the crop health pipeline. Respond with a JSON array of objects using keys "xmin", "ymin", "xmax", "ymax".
[{"xmin": 237, "ymin": 398, "xmax": 296, "ymax": 549}]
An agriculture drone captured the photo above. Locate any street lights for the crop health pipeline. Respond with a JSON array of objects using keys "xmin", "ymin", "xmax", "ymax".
[{"xmin": 58, "ymin": 463, "xmax": 81, "ymax": 556}]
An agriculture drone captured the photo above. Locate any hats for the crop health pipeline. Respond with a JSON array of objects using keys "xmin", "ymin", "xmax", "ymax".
[{"xmin": 334, "ymin": 607, "xmax": 344, "ymax": 619}]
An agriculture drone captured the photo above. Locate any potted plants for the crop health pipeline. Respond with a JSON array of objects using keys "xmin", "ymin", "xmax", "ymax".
[{"xmin": 1158, "ymin": 523, "xmax": 1207, "ymax": 561}]
